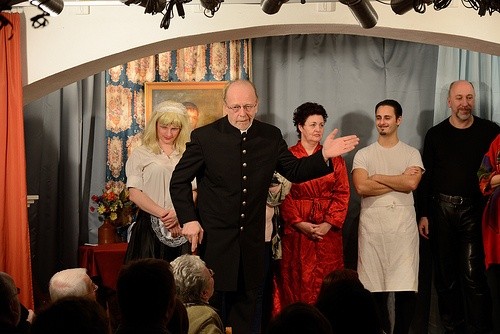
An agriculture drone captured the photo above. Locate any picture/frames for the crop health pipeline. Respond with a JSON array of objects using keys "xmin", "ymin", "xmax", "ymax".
[{"xmin": 144, "ymin": 81, "xmax": 230, "ymax": 132}]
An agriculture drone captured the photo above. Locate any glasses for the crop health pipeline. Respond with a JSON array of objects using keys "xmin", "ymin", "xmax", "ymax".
[
  {"xmin": 87, "ymin": 284, "xmax": 99, "ymax": 296},
  {"xmin": 206, "ymin": 268, "xmax": 215, "ymax": 281},
  {"xmin": 225, "ymin": 101, "xmax": 258, "ymax": 111}
]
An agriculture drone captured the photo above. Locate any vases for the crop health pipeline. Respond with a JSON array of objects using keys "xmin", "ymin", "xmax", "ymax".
[{"xmin": 98, "ymin": 219, "xmax": 114, "ymax": 245}]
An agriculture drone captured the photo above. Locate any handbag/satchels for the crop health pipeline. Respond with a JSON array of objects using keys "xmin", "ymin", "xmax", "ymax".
[{"xmin": 270, "ymin": 215, "xmax": 283, "ymax": 261}]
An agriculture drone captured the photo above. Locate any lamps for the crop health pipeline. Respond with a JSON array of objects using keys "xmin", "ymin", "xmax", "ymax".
[
  {"xmin": 391, "ymin": 0, "xmax": 432, "ymax": 15},
  {"xmin": 120, "ymin": 0, "xmax": 224, "ymax": 30},
  {"xmin": 337, "ymin": 0, "xmax": 378, "ymax": 30},
  {"xmin": 0, "ymin": 0, "xmax": 64, "ymax": 40},
  {"xmin": 260, "ymin": 0, "xmax": 306, "ymax": 15}
]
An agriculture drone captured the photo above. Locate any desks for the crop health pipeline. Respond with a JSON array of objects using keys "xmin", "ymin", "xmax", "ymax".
[{"xmin": 80, "ymin": 243, "xmax": 128, "ymax": 319}]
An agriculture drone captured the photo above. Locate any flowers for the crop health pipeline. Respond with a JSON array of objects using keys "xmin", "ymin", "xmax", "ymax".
[{"xmin": 90, "ymin": 180, "xmax": 130, "ymax": 221}]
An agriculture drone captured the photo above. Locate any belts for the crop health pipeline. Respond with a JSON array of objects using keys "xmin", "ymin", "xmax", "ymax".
[{"xmin": 438, "ymin": 193, "xmax": 474, "ymax": 206}]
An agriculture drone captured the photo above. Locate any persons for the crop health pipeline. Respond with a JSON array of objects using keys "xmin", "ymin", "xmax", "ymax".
[
  {"xmin": 0, "ymin": 253, "xmax": 222, "ymax": 334},
  {"xmin": 411, "ymin": 80, "xmax": 500, "ymax": 334},
  {"xmin": 169, "ymin": 79, "xmax": 359, "ymax": 334},
  {"xmin": 122, "ymin": 100, "xmax": 198, "ymax": 265},
  {"xmin": 272, "ymin": 102, "xmax": 350, "ymax": 321},
  {"xmin": 268, "ymin": 269, "xmax": 383, "ymax": 334},
  {"xmin": 477, "ymin": 135, "xmax": 500, "ymax": 334},
  {"xmin": 350, "ymin": 100, "xmax": 426, "ymax": 334}
]
